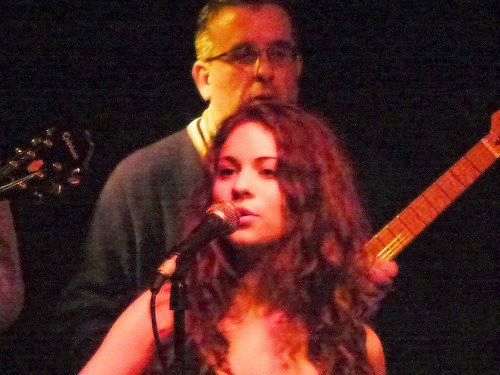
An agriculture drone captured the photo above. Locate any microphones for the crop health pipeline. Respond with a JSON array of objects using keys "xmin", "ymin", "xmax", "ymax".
[{"xmin": 150, "ymin": 202, "xmax": 240, "ymax": 295}]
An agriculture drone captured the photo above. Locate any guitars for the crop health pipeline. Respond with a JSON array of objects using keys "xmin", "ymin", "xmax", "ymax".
[
  {"xmin": 362, "ymin": 109, "xmax": 500, "ymax": 263},
  {"xmin": 0, "ymin": 125, "xmax": 95, "ymax": 206}
]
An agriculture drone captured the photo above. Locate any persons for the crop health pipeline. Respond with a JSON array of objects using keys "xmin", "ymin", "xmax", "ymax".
[
  {"xmin": 74, "ymin": 0, "xmax": 398, "ymax": 369},
  {"xmin": 74, "ymin": 102, "xmax": 387, "ymax": 375},
  {"xmin": 0, "ymin": 201, "xmax": 25, "ymax": 334}
]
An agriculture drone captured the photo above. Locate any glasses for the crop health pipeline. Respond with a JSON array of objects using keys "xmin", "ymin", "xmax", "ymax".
[{"xmin": 206, "ymin": 41, "xmax": 301, "ymax": 66}]
